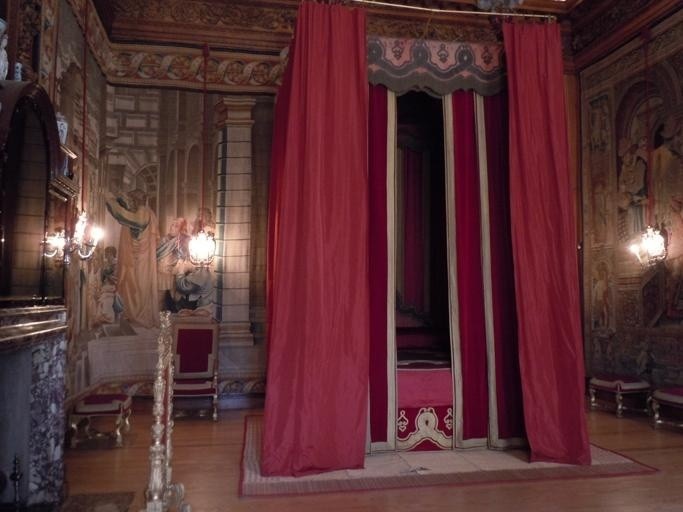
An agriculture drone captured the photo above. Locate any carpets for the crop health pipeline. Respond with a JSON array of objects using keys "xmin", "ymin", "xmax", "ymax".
[{"xmin": 237, "ymin": 413, "xmax": 659, "ymax": 500}]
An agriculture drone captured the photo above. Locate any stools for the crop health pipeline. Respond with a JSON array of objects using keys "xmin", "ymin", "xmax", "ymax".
[
  {"xmin": 67, "ymin": 394, "xmax": 132, "ymax": 449},
  {"xmin": 588, "ymin": 372, "xmax": 682, "ymax": 432}
]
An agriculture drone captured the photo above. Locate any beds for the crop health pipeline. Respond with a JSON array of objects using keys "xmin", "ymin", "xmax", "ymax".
[{"xmin": 394, "ymin": 289, "xmax": 453, "ymax": 453}]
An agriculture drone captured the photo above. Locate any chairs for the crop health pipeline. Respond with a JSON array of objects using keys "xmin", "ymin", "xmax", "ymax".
[{"xmin": 165, "ymin": 323, "xmax": 219, "ymax": 421}]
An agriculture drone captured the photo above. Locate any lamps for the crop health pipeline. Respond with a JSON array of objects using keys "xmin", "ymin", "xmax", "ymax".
[
  {"xmin": 628, "ymin": 0, "xmax": 667, "ymax": 266},
  {"xmin": 72, "ymin": 0, "xmax": 104, "ymax": 261},
  {"xmin": 41, "ymin": 228, "xmax": 70, "ymax": 260},
  {"xmin": 187, "ymin": 43, "xmax": 217, "ymax": 264}
]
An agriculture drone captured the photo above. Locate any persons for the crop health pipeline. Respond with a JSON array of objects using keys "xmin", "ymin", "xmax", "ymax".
[
  {"xmin": 590, "ymin": 269, "xmax": 613, "ymax": 327},
  {"xmin": 618, "ymin": 144, "xmax": 650, "ymax": 238},
  {"xmin": 93, "ymin": 186, "xmax": 217, "ymax": 329}
]
